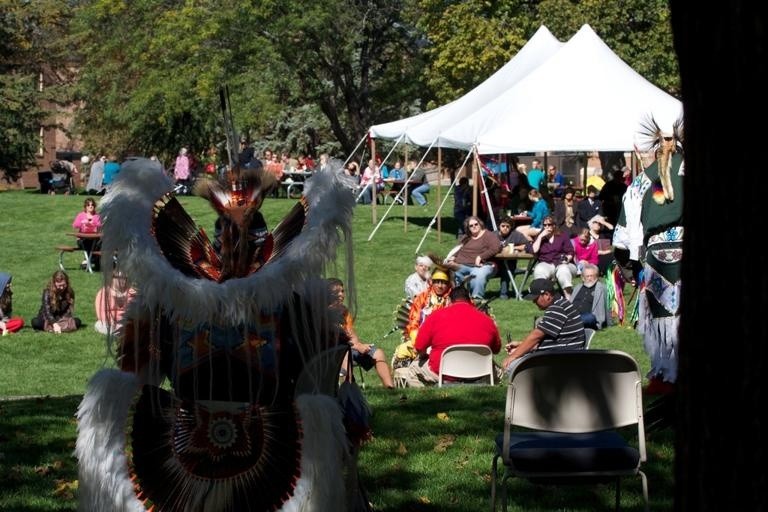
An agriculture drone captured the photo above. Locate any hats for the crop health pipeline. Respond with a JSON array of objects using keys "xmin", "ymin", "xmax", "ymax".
[
  {"xmin": 523, "ymin": 279, "xmax": 554, "ymax": 300},
  {"xmin": 430, "ymin": 269, "xmax": 450, "ymax": 282}
]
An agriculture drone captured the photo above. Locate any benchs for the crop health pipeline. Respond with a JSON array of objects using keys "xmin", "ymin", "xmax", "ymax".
[
  {"xmin": 94, "ymin": 251, "xmax": 119, "ymax": 273},
  {"xmin": 280, "ymin": 181, "xmax": 305, "ymax": 200},
  {"xmin": 54, "ymin": 246, "xmax": 81, "ymax": 272},
  {"xmin": 380, "ymin": 190, "xmax": 398, "ymax": 205},
  {"xmin": 485, "ymin": 266, "xmax": 534, "ymax": 280}
]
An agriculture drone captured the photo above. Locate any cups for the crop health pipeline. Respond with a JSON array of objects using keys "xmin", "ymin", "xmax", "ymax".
[
  {"xmin": 508, "ymin": 242, "xmax": 515, "ymax": 256},
  {"xmin": 290, "ymin": 165, "xmax": 295, "ymax": 172}
]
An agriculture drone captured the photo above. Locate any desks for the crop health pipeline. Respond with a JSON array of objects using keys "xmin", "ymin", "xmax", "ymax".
[
  {"xmin": 66, "ymin": 233, "xmax": 101, "ymax": 274},
  {"xmin": 281, "ymin": 170, "xmax": 312, "ymax": 179},
  {"xmin": 383, "ymin": 179, "xmax": 422, "ymax": 197},
  {"xmin": 494, "ymin": 253, "xmax": 537, "ymax": 303},
  {"xmin": 510, "ymin": 215, "xmax": 532, "ymax": 229}
]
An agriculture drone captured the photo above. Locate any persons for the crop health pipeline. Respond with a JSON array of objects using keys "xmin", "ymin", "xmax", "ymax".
[
  {"xmin": 326, "ymin": 279, "xmax": 395, "ymax": 390},
  {"xmin": 392, "ymin": 159, "xmax": 638, "ymax": 389},
  {"xmin": 74, "ymin": 198, "xmax": 103, "ymax": 268},
  {"xmin": 30, "ymin": 270, "xmax": 81, "ymax": 334},
  {"xmin": 74, "ymin": 154, "xmax": 372, "ymax": 509},
  {"xmin": 173, "ymin": 147, "xmax": 194, "ymax": 193},
  {"xmin": 1, "ymin": 272, "xmax": 24, "ymax": 335},
  {"xmin": 237, "ymin": 140, "xmax": 432, "ymax": 206},
  {"xmin": 49, "ymin": 152, "xmax": 120, "ymax": 196},
  {"xmin": 94, "ymin": 269, "xmax": 136, "ymax": 336}
]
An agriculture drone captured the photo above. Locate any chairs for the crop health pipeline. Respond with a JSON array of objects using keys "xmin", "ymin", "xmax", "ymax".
[
  {"xmin": 584, "ymin": 328, "xmax": 596, "ymax": 350},
  {"xmin": 352, "ymin": 360, "xmax": 365, "ymax": 390},
  {"xmin": 491, "ymin": 349, "xmax": 649, "ymax": 512},
  {"xmin": 435, "ymin": 344, "xmax": 495, "ymax": 391}
]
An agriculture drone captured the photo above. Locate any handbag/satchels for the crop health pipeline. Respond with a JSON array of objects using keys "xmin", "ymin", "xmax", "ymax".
[
  {"xmin": 338, "ymin": 382, "xmax": 373, "ymax": 440},
  {"xmin": 44, "ymin": 317, "xmax": 77, "ymax": 332}
]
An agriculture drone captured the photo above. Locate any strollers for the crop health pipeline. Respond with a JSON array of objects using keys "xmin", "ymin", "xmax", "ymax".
[{"xmin": 49, "ymin": 158, "xmax": 77, "ymax": 196}]
